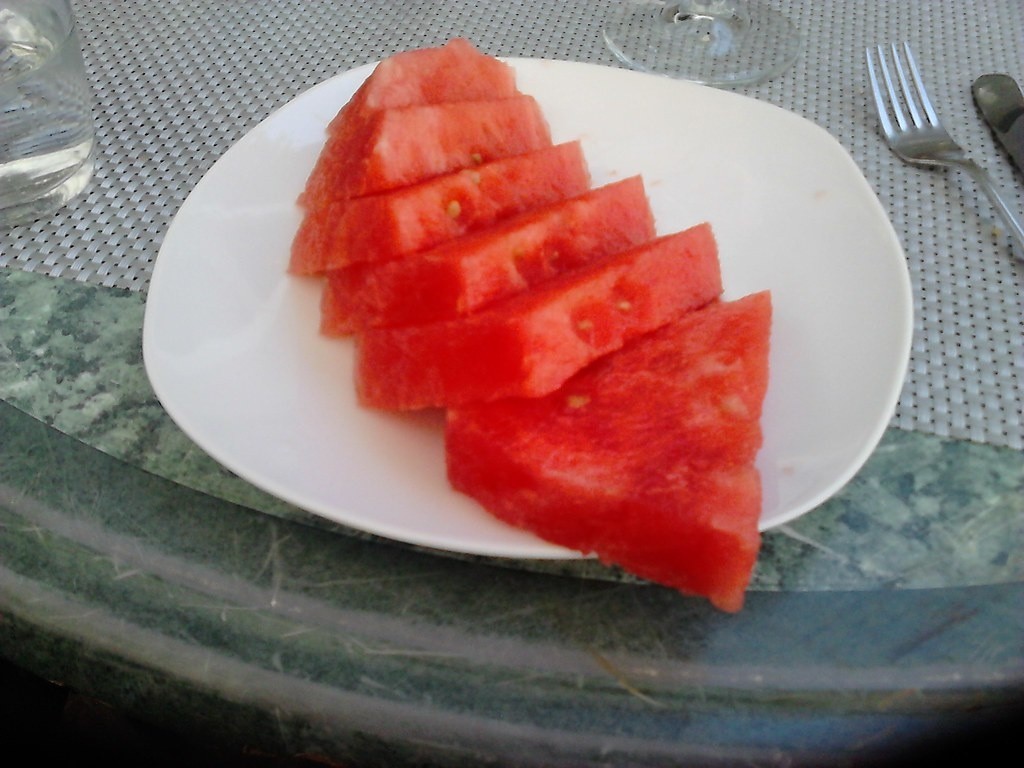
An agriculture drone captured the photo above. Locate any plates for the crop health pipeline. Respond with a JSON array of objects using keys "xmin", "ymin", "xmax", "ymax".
[{"xmin": 143, "ymin": 57, "xmax": 913, "ymax": 559}]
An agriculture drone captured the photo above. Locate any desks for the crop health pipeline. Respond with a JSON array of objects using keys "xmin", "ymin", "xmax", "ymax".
[{"xmin": 0, "ymin": 0, "xmax": 1024, "ymax": 768}]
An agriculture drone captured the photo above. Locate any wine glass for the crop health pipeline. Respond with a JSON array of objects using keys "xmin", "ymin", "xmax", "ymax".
[{"xmin": 602, "ymin": 0, "xmax": 803, "ymax": 88}]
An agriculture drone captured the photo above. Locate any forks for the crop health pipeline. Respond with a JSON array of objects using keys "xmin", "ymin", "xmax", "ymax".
[{"xmin": 865, "ymin": 42, "xmax": 1024, "ymax": 248}]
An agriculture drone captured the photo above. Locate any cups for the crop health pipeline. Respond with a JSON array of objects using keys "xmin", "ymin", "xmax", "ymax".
[{"xmin": 0, "ymin": 0, "xmax": 97, "ymax": 229}]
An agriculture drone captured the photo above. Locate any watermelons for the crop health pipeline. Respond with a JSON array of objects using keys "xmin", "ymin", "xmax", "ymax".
[{"xmin": 291, "ymin": 39, "xmax": 771, "ymax": 605}]
